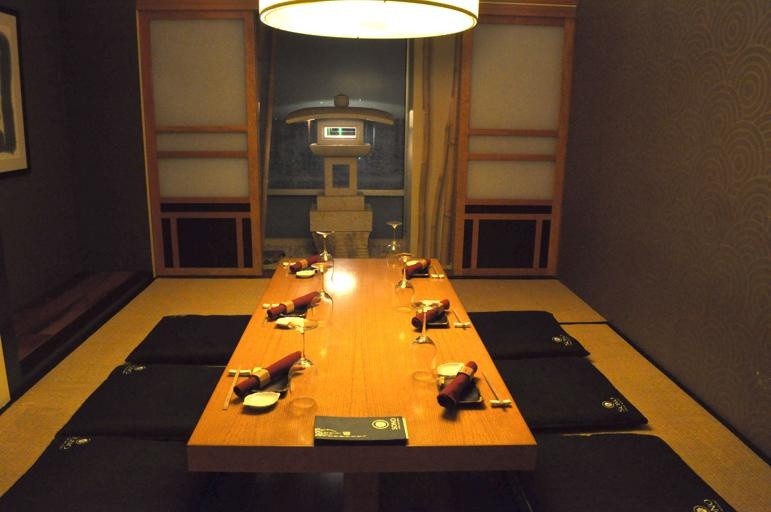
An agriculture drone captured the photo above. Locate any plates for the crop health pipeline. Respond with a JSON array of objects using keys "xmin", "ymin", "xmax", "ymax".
[
  {"xmin": 275, "ymin": 316, "xmax": 308, "ymax": 327},
  {"xmin": 242, "ymin": 391, "xmax": 280, "ymax": 409},
  {"xmin": 296, "ymin": 269, "xmax": 316, "ymax": 278},
  {"xmin": 436, "ymin": 362, "xmax": 464, "ymax": 378}
]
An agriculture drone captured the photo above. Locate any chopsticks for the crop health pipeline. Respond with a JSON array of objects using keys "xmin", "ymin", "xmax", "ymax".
[
  {"xmin": 481, "ymin": 371, "xmax": 505, "ymax": 406},
  {"xmin": 452, "ymin": 307, "xmax": 466, "ymax": 328},
  {"xmin": 222, "ymin": 367, "xmax": 240, "ymax": 410},
  {"xmin": 431, "ymin": 263, "xmax": 440, "ymax": 280}
]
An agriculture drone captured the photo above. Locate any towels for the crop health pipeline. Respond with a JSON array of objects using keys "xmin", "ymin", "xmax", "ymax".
[
  {"xmin": 291, "ymin": 255, "xmax": 317, "ymax": 271},
  {"xmin": 412, "ymin": 300, "xmax": 450, "ymax": 326},
  {"xmin": 267, "ymin": 291, "xmax": 320, "ymax": 318},
  {"xmin": 234, "ymin": 351, "xmax": 301, "ymax": 396},
  {"xmin": 437, "ymin": 362, "xmax": 478, "ymax": 407}
]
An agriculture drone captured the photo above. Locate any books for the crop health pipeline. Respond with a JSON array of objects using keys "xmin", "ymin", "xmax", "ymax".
[{"xmin": 314, "ymin": 415, "xmax": 409, "ymax": 445}]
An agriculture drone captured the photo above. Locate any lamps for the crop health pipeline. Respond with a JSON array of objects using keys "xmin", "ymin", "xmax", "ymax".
[{"xmin": 256, "ymin": 1, "xmax": 486, "ymax": 41}]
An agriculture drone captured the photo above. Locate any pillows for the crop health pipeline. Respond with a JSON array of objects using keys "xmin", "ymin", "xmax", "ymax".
[{"xmin": 403, "ymin": 260, "xmax": 431, "ymax": 276}]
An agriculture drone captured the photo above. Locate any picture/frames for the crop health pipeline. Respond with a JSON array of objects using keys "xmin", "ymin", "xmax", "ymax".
[{"xmin": 0, "ymin": 14, "xmax": 29, "ymax": 174}]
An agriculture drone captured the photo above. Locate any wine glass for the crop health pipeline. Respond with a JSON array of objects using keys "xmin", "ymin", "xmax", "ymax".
[
  {"xmin": 311, "ymin": 262, "xmax": 334, "ymax": 327},
  {"xmin": 287, "ymin": 319, "xmax": 320, "ymax": 412},
  {"xmin": 405, "ymin": 300, "xmax": 441, "ymax": 381},
  {"xmin": 316, "ymin": 230, "xmax": 336, "ymax": 281},
  {"xmin": 384, "ymin": 221, "xmax": 402, "ymax": 275},
  {"xmin": 394, "ymin": 252, "xmax": 416, "ymax": 312}
]
